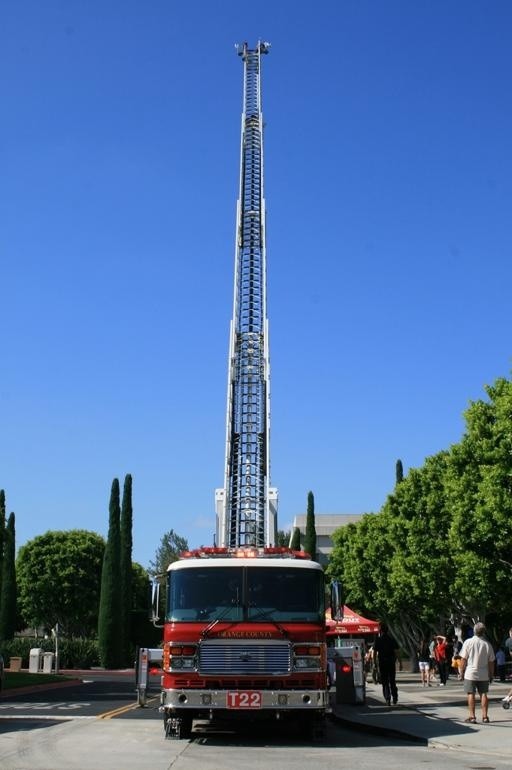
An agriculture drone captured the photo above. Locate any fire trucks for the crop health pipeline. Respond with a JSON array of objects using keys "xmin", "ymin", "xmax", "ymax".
[{"xmin": 134, "ymin": 544, "xmax": 366, "ymax": 745}]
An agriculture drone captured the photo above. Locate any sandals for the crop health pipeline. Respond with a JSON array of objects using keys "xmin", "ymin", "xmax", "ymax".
[
  {"xmin": 483, "ymin": 718, "xmax": 489, "ymax": 723},
  {"xmin": 464, "ymin": 717, "xmax": 476, "ymax": 723}
]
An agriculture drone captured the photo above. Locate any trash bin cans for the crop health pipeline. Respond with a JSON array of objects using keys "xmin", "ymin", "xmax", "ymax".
[{"xmin": 29, "ymin": 648, "xmax": 54, "ymax": 674}]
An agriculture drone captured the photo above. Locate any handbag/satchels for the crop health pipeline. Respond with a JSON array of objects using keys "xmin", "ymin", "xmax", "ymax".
[{"xmin": 439, "ymin": 658, "xmax": 444, "ymax": 665}]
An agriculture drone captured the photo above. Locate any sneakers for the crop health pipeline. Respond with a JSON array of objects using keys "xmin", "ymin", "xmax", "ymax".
[
  {"xmin": 421, "ymin": 684, "xmax": 432, "ymax": 687},
  {"xmin": 502, "ymin": 696, "xmax": 512, "ymax": 702},
  {"xmin": 385, "ymin": 699, "xmax": 397, "ymax": 706}
]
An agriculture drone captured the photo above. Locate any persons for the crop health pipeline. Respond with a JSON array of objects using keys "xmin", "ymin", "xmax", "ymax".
[
  {"xmin": 364, "ymin": 622, "xmax": 403, "ymax": 706},
  {"xmin": 416, "ymin": 621, "xmax": 512, "ymax": 724}
]
